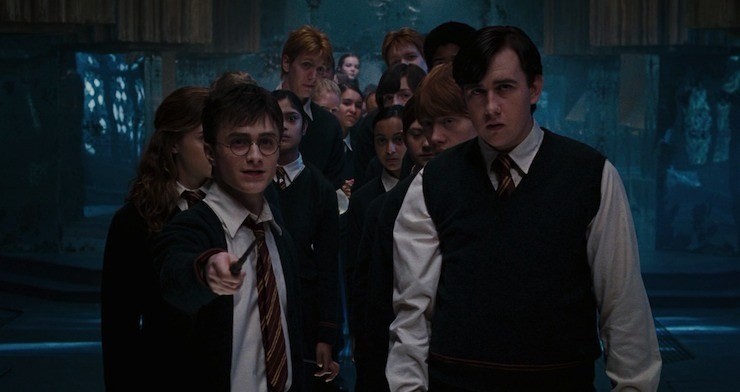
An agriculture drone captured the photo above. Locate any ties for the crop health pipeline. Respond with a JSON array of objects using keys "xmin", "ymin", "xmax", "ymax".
[
  {"xmin": 181, "ymin": 191, "xmax": 206, "ymax": 209},
  {"xmin": 275, "ymin": 167, "xmax": 286, "ymax": 190},
  {"xmin": 242, "ymin": 217, "xmax": 289, "ymax": 392},
  {"xmin": 497, "ymin": 155, "xmax": 515, "ymax": 196}
]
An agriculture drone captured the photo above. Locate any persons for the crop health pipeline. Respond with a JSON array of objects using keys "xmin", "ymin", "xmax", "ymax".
[
  {"xmin": 101, "ymin": 21, "xmax": 478, "ymax": 392},
  {"xmin": 386, "ymin": 24, "xmax": 662, "ymax": 392}
]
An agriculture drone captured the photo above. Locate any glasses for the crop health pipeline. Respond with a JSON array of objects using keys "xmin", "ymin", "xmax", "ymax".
[{"xmin": 215, "ymin": 135, "xmax": 279, "ymax": 156}]
